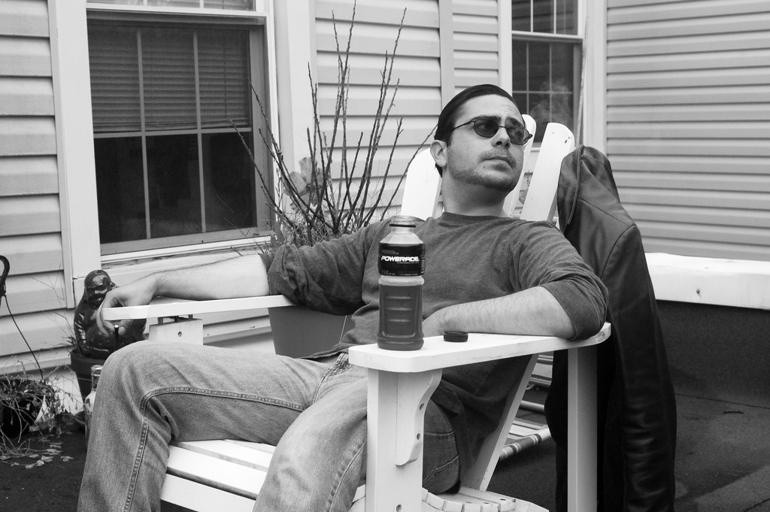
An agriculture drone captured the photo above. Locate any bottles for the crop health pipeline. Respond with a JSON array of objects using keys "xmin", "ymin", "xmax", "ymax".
[
  {"xmin": 378, "ymin": 213, "xmax": 424, "ymax": 351},
  {"xmin": 84, "ymin": 365, "xmax": 104, "ymax": 452}
]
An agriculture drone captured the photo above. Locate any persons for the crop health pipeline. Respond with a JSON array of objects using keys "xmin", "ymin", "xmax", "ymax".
[
  {"xmin": 75, "ymin": 83, "xmax": 611, "ymax": 511},
  {"xmin": 74, "ymin": 270, "xmax": 147, "ymax": 358}
]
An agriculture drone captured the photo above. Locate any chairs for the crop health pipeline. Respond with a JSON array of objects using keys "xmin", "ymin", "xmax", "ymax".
[{"xmin": 101, "ymin": 113, "xmax": 636, "ymax": 510}]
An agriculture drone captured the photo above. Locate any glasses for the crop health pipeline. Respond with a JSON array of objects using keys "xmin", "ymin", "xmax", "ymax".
[{"xmin": 454, "ymin": 116, "xmax": 533, "ymax": 146}]
